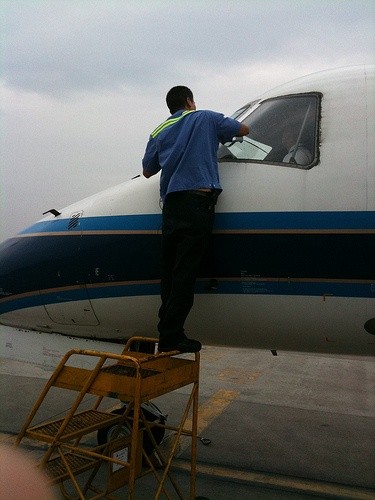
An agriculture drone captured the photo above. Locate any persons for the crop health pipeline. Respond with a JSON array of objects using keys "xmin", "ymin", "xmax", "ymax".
[
  {"xmin": 142, "ymin": 86, "xmax": 250, "ymax": 353},
  {"xmin": 281, "ymin": 120, "xmax": 313, "ymax": 166}
]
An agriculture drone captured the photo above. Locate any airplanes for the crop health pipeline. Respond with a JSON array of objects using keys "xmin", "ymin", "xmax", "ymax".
[{"xmin": 0, "ymin": 63, "xmax": 374, "ymax": 500}]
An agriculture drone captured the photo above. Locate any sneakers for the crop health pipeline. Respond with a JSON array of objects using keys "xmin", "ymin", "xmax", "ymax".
[{"xmin": 159, "ymin": 334, "xmax": 201, "ymax": 353}]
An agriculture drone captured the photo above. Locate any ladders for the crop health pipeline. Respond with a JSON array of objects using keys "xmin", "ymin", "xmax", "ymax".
[{"xmin": 1, "ymin": 336, "xmax": 201, "ymax": 499}]
